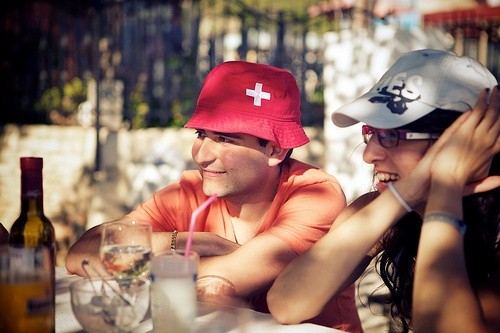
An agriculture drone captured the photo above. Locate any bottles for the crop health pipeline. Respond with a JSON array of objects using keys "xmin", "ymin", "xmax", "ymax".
[{"xmin": 0, "ymin": 156, "xmax": 55, "ymax": 333}]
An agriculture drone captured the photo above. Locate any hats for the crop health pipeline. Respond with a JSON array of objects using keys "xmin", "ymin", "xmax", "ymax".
[
  {"xmin": 331, "ymin": 48, "xmax": 498, "ymax": 130},
  {"xmin": 183, "ymin": 60, "xmax": 310, "ymax": 149}
]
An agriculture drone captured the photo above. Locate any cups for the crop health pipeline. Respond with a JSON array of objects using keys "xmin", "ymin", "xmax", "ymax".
[{"xmin": 148, "ymin": 250, "xmax": 199, "ymax": 333}]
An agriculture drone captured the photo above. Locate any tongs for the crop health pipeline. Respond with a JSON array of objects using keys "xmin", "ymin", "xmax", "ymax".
[{"xmin": 82, "ymin": 260, "xmax": 133, "ymax": 325}]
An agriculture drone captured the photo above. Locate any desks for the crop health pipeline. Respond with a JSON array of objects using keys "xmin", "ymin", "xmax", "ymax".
[{"xmin": 55, "ymin": 267, "xmax": 349, "ymax": 333}]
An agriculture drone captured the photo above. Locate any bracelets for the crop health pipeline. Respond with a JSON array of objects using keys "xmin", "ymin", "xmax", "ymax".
[
  {"xmin": 170, "ymin": 230, "xmax": 177, "ymax": 253},
  {"xmin": 388, "ymin": 181, "xmax": 412, "ymax": 212},
  {"xmin": 423, "ymin": 213, "xmax": 467, "ymax": 233}
]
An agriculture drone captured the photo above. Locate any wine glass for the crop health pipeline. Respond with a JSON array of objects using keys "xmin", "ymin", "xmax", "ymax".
[{"xmin": 99, "ymin": 222, "xmax": 152, "ymax": 294}]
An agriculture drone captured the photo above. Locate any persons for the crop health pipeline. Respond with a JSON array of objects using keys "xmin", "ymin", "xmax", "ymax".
[
  {"xmin": 65, "ymin": 61, "xmax": 365, "ymax": 333},
  {"xmin": 266, "ymin": 49, "xmax": 500, "ymax": 333}
]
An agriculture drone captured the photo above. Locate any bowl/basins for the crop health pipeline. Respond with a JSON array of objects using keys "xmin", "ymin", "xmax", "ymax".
[{"xmin": 69, "ymin": 276, "xmax": 151, "ymax": 333}]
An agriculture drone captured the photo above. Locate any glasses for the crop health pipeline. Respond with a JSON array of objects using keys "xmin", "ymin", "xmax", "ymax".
[{"xmin": 362, "ymin": 124, "xmax": 441, "ymax": 149}]
